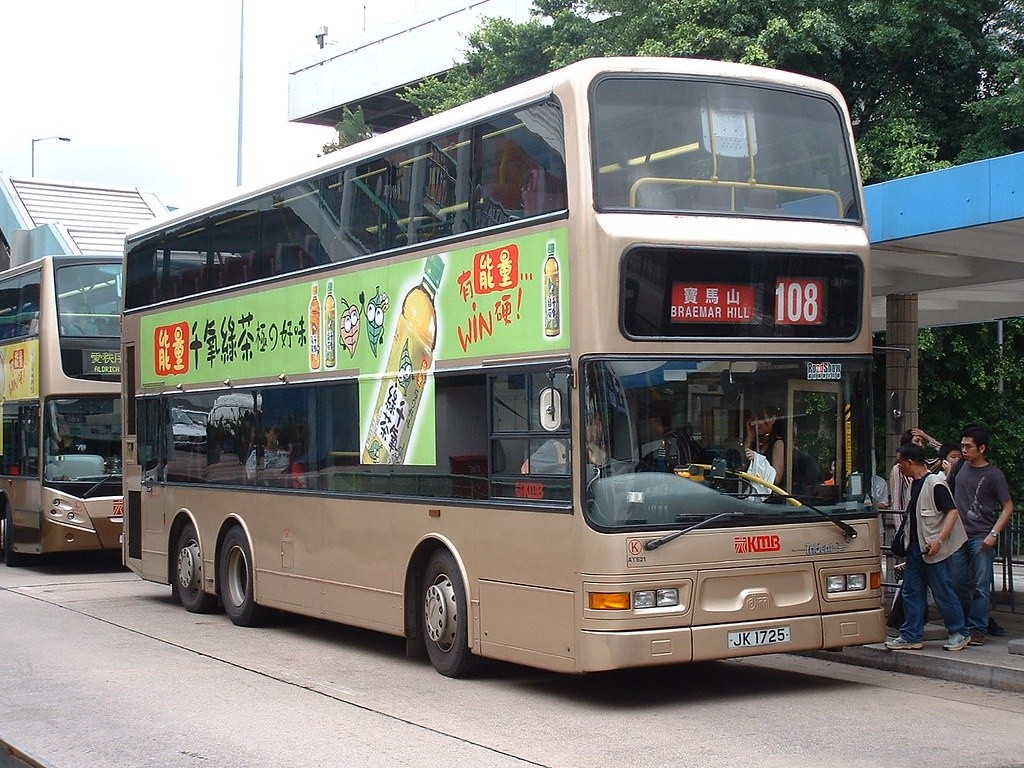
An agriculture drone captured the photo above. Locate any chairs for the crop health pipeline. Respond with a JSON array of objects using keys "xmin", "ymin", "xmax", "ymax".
[{"xmin": 743, "ymin": 186, "xmax": 786, "ymax": 217}]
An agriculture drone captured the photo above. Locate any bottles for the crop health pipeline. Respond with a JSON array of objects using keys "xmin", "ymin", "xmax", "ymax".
[
  {"xmin": 309, "ymin": 286, "xmax": 321, "ymax": 370},
  {"xmin": 324, "ymin": 282, "xmax": 337, "ymax": 369},
  {"xmin": 544, "ymin": 243, "xmax": 561, "ymax": 336},
  {"xmin": 361, "ymin": 253, "xmax": 446, "ymax": 467},
  {"xmin": 30, "ymin": 353, "xmax": 34, "ymax": 394}
]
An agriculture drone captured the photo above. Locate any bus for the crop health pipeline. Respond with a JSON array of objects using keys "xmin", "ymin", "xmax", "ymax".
[
  {"xmin": 0, "ymin": 255, "xmax": 220, "ymax": 568},
  {"xmin": 121, "ymin": 58, "xmax": 913, "ymax": 680}
]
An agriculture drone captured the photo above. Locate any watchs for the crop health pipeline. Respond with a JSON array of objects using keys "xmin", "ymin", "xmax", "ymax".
[{"xmin": 991, "ymin": 530, "xmax": 998, "ymax": 538}]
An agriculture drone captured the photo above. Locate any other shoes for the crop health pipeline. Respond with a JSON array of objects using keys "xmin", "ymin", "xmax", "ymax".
[
  {"xmin": 968, "ymin": 629, "xmax": 986, "ymax": 645},
  {"xmin": 899, "ymin": 619, "xmax": 927, "ymax": 631},
  {"xmin": 987, "ymin": 617, "xmax": 1004, "ymax": 633}
]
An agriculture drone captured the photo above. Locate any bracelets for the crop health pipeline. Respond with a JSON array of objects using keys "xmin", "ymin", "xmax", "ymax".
[{"xmin": 937, "ymin": 537, "xmax": 944, "ymax": 545}]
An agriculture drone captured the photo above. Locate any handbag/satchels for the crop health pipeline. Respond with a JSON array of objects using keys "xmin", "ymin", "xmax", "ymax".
[{"xmin": 891, "ymin": 530, "xmax": 907, "ymax": 557}]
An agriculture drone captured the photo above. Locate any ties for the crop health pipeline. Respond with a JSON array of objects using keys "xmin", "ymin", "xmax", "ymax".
[{"xmin": 586, "ymin": 447, "xmax": 590, "ymax": 464}]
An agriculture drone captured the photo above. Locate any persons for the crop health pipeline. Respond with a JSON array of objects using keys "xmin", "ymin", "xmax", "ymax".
[
  {"xmin": 58, "ymin": 422, "xmax": 86, "ymax": 454},
  {"xmin": 520, "ymin": 409, "xmax": 610, "ymax": 473},
  {"xmin": 872, "ymin": 424, "xmax": 1014, "ymax": 651},
  {"xmin": 741, "ymin": 402, "xmax": 786, "ymax": 504},
  {"xmin": 245, "ymin": 422, "xmax": 307, "ymax": 490},
  {"xmin": 792, "ymin": 422, "xmax": 825, "ymax": 488},
  {"xmin": 824, "ymin": 455, "xmax": 836, "ymax": 486}
]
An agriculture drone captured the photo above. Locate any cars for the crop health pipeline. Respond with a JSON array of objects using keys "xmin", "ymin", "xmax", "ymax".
[{"xmin": 172, "ymin": 408, "xmax": 209, "ymax": 455}]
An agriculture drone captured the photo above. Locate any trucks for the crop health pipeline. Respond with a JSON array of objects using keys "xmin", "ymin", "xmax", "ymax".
[{"xmin": 208, "ymin": 395, "xmax": 263, "ymax": 435}]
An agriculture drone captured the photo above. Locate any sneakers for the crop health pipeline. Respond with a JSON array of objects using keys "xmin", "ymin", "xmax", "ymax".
[
  {"xmin": 884, "ymin": 636, "xmax": 923, "ymax": 649},
  {"xmin": 943, "ymin": 632, "xmax": 971, "ymax": 650}
]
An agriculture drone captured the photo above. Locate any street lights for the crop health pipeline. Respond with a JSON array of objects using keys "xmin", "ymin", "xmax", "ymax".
[{"xmin": 32, "ymin": 137, "xmax": 71, "ymax": 178}]
